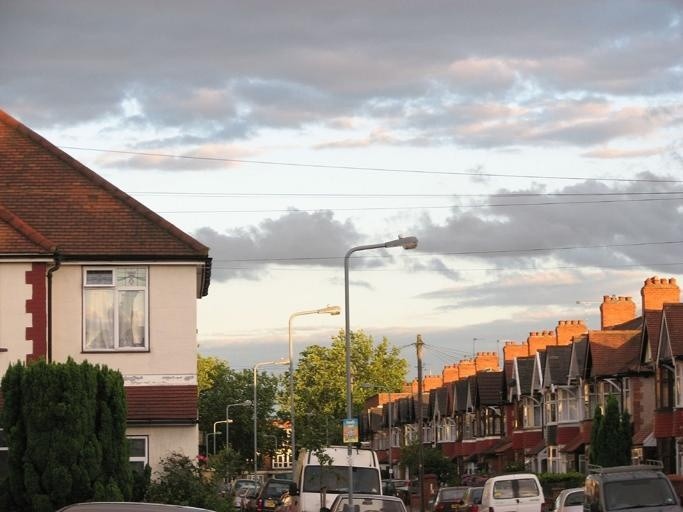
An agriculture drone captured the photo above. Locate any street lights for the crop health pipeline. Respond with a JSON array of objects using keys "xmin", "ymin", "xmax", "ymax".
[
  {"xmin": 206, "ymin": 399, "xmax": 252, "ymax": 460},
  {"xmin": 287, "ymin": 306, "xmax": 341, "ymax": 467},
  {"xmin": 361, "ymin": 383, "xmax": 392, "ymax": 478},
  {"xmin": 263, "ymin": 434, "xmax": 278, "ymax": 453},
  {"xmin": 253, "ymin": 360, "xmax": 291, "ymax": 476},
  {"xmin": 345, "ymin": 236, "xmax": 418, "ymax": 512}
]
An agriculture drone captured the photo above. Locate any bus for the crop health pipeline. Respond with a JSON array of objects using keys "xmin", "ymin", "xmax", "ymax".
[{"xmin": 294, "ymin": 445, "xmax": 384, "ymax": 512}]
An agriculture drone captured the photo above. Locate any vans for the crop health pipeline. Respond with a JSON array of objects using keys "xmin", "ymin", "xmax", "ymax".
[
  {"xmin": 474, "ymin": 473, "xmax": 545, "ymax": 512},
  {"xmin": 585, "ymin": 459, "xmax": 682, "ymax": 512}
]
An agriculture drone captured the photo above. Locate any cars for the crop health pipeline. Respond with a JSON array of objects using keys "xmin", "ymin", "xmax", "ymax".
[
  {"xmin": 321, "ymin": 493, "xmax": 409, "ymax": 512},
  {"xmin": 428, "ymin": 487, "xmax": 484, "ymax": 512},
  {"xmin": 382, "ymin": 478, "xmax": 416, "ymax": 494},
  {"xmin": 549, "ymin": 489, "xmax": 586, "ymax": 512}
]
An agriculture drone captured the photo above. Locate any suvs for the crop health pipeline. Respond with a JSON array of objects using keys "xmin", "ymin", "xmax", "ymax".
[{"xmin": 232, "ymin": 478, "xmax": 299, "ymax": 511}]
